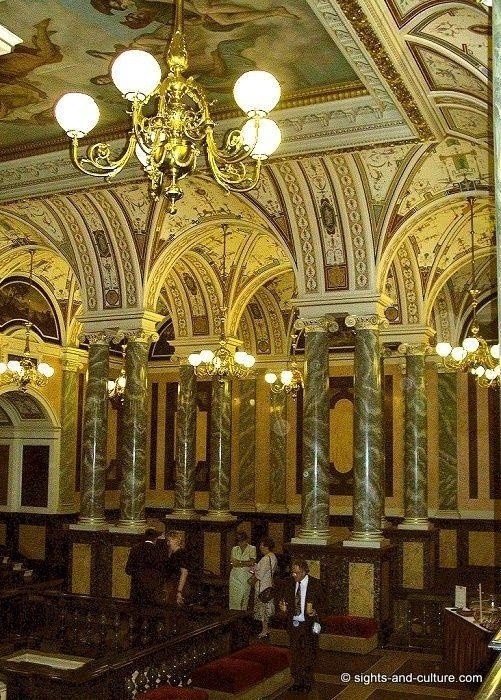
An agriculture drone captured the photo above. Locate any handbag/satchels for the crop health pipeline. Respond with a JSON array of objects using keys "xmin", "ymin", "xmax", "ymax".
[{"xmin": 258, "ymin": 587, "xmax": 276, "ymax": 603}]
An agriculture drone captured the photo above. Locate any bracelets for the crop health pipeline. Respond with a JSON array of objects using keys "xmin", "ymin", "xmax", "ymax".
[{"xmin": 177, "ymin": 590, "xmax": 182, "ymax": 593}]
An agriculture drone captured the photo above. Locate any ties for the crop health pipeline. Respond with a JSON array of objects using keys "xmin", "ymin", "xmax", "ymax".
[{"xmin": 294, "ymin": 581, "xmax": 301, "ymax": 616}]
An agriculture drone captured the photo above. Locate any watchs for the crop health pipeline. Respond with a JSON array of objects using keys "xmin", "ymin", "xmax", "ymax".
[{"xmin": 239, "ymin": 561, "xmax": 242, "ymax": 566}]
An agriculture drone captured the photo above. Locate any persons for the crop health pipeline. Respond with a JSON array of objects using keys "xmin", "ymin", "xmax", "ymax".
[
  {"xmin": 273, "ymin": 559, "xmax": 328, "ymax": 697},
  {"xmin": 125, "ymin": 528, "xmax": 164, "ymax": 600},
  {"xmin": 252, "ymin": 536, "xmax": 277, "ymax": 639},
  {"xmin": 85, "ymin": 0, "xmax": 300, "ymax": 94},
  {"xmin": 0, "ymin": 19, "xmax": 64, "ymax": 118},
  {"xmin": 228, "ymin": 530, "xmax": 257, "ymax": 611},
  {"xmin": 165, "ymin": 531, "xmax": 189, "ymax": 606}
]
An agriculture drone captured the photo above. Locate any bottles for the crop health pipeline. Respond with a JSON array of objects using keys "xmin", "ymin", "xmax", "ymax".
[
  {"xmin": 305, "ymin": 595, "xmax": 314, "ymax": 614},
  {"xmin": 279, "ymin": 597, "xmax": 287, "ymax": 612}
]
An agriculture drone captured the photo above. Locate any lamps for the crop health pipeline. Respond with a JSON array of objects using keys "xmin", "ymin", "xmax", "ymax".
[
  {"xmin": 0, "ymin": 0, "xmax": 500, "ymax": 405},
  {"xmin": 53, "ymin": 0, "xmax": 283, "ymax": 214}
]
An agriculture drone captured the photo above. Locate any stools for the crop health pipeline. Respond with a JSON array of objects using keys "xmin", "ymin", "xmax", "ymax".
[{"xmin": 133, "ymin": 612, "xmax": 380, "ymax": 700}]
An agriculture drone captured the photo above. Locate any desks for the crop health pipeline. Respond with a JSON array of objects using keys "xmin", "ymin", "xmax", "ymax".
[{"xmin": 445, "ymin": 606, "xmax": 501, "ymax": 685}]
